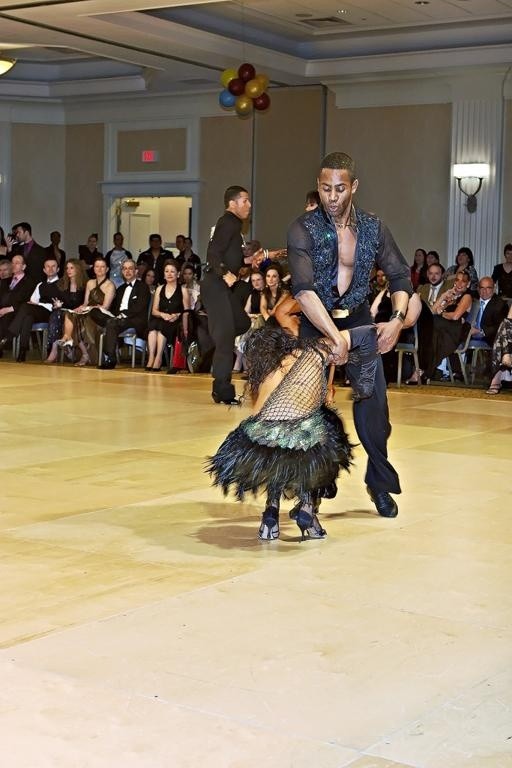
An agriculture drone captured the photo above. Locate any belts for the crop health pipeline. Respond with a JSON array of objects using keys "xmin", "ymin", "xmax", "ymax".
[{"xmin": 331, "ymin": 309, "xmax": 350, "ymax": 319}]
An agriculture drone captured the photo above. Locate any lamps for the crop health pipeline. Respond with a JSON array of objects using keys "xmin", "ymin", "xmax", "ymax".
[
  {"xmin": 452, "ymin": 163, "xmax": 490, "ymax": 213},
  {"xmin": 0, "ymin": 58, "xmax": 17, "ymax": 76}
]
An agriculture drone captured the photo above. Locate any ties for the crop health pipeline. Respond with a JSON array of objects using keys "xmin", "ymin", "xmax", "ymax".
[
  {"xmin": 428, "ymin": 287, "xmax": 436, "ymax": 306},
  {"xmin": 474, "ymin": 301, "xmax": 485, "ymax": 329}
]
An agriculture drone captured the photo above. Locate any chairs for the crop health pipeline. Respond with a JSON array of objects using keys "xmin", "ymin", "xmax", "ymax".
[
  {"xmin": 394, "ymin": 297, "xmax": 512, "ymax": 390},
  {"xmin": 12, "ymin": 294, "xmax": 174, "ymax": 370}
]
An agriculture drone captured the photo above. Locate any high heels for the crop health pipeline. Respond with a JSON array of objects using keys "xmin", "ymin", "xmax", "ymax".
[
  {"xmin": 56, "ymin": 335, "xmax": 74, "ymax": 347},
  {"xmin": 485, "ymin": 382, "xmax": 502, "ymax": 395},
  {"xmin": 74, "ymin": 354, "xmax": 91, "ymax": 367},
  {"xmin": 405, "ymin": 371, "xmax": 425, "ymax": 385},
  {"xmin": 257, "ymin": 506, "xmax": 280, "ymax": 543},
  {"xmin": 297, "ymin": 510, "xmax": 327, "ymax": 541},
  {"xmin": 44, "ymin": 353, "xmax": 58, "ymax": 363},
  {"xmin": 146, "ymin": 361, "xmax": 250, "ymax": 374}
]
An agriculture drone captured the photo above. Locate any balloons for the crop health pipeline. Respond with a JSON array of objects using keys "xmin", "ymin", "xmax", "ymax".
[{"xmin": 218, "ymin": 62, "xmax": 270, "ymax": 119}]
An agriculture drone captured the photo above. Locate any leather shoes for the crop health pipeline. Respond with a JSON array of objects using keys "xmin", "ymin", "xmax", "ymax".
[
  {"xmin": 0, "ymin": 335, "xmax": 10, "ymax": 345},
  {"xmin": 97, "ymin": 357, "xmax": 116, "ymax": 369},
  {"xmin": 320, "ymin": 481, "xmax": 337, "ymax": 498},
  {"xmin": 212, "ymin": 391, "xmax": 240, "ymax": 405},
  {"xmin": 367, "ymin": 484, "xmax": 398, "ymax": 518},
  {"xmin": 18, "ymin": 351, "xmax": 26, "ymax": 362}
]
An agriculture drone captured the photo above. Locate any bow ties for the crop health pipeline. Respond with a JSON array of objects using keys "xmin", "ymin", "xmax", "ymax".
[{"xmin": 124, "ymin": 282, "xmax": 133, "ymax": 288}]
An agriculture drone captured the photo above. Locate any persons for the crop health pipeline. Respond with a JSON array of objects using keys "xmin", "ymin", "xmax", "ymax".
[
  {"xmin": 0, "ymin": 222, "xmax": 218, "ymax": 375},
  {"xmin": 289, "ymin": 150, "xmax": 410, "ymax": 518},
  {"xmin": 333, "ymin": 246, "xmax": 511, "ymax": 395},
  {"xmin": 199, "ymin": 185, "xmax": 289, "ymax": 404},
  {"xmin": 231, "ymin": 190, "xmax": 322, "ymax": 376},
  {"xmin": 203, "ymin": 322, "xmax": 392, "ymax": 542}
]
